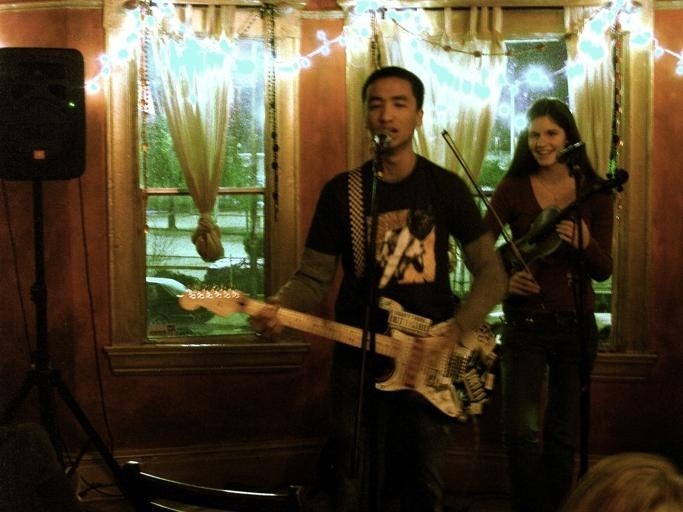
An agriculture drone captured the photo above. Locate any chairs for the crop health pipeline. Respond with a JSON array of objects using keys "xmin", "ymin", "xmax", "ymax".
[{"xmin": 120, "ymin": 461, "xmax": 307, "ymax": 510}]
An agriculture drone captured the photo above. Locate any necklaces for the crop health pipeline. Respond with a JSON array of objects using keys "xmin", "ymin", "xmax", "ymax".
[{"xmin": 537, "ymin": 174, "xmax": 574, "ymax": 202}]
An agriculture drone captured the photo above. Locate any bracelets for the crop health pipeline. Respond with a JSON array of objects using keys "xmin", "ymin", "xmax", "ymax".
[{"xmin": 452, "ymin": 314, "xmax": 464, "ymax": 334}]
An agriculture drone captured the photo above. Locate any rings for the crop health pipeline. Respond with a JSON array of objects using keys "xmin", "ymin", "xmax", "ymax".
[{"xmin": 255, "ymin": 330, "xmax": 262, "ymax": 336}]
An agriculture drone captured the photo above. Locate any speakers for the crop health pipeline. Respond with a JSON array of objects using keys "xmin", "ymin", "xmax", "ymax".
[{"xmin": 0, "ymin": 47, "xmax": 86, "ymax": 182}]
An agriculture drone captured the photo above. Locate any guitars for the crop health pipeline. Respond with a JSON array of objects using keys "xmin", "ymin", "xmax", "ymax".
[{"xmin": 180, "ymin": 289, "xmax": 496, "ymax": 423}]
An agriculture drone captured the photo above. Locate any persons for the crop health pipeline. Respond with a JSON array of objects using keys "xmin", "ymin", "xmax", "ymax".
[
  {"xmin": 566, "ymin": 453, "xmax": 682, "ymax": 511},
  {"xmin": 482, "ymin": 97, "xmax": 615, "ymax": 508},
  {"xmin": 249, "ymin": 65, "xmax": 508, "ymax": 487}
]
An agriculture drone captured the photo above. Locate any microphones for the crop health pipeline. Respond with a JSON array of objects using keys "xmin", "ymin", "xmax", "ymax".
[
  {"xmin": 556, "ymin": 141, "xmax": 586, "ymax": 174},
  {"xmin": 373, "ymin": 132, "xmax": 392, "ymax": 152}
]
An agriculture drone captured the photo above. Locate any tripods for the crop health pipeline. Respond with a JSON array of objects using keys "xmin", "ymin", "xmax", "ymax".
[{"xmin": 0, "ymin": 182, "xmax": 122, "ymax": 483}]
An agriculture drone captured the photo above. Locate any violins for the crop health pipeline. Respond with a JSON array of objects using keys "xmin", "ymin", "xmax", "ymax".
[{"xmin": 499, "ymin": 169, "xmax": 628, "ymax": 280}]
